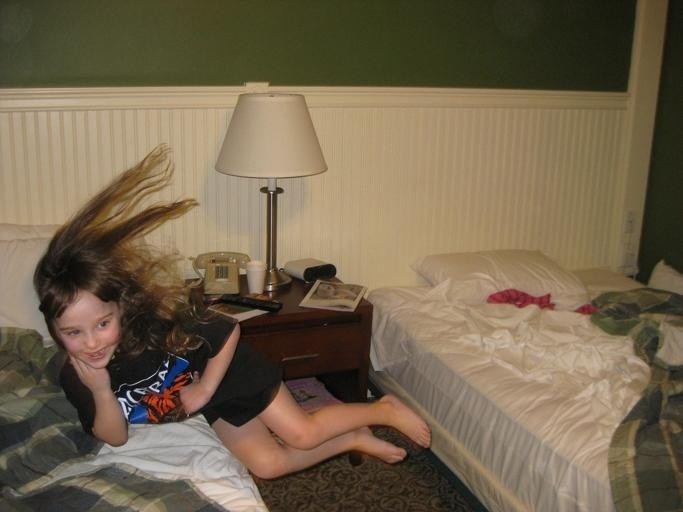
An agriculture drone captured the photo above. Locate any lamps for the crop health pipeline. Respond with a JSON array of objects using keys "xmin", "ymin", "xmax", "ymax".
[{"xmin": 214, "ymin": 93, "xmax": 329, "ymax": 292}]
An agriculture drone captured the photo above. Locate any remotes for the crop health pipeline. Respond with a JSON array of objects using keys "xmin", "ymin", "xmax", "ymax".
[{"xmin": 219, "ymin": 294, "xmax": 283, "ymax": 312}]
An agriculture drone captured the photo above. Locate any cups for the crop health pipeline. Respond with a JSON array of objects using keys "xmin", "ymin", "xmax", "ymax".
[{"xmin": 246, "ymin": 261, "xmax": 265, "ymax": 294}]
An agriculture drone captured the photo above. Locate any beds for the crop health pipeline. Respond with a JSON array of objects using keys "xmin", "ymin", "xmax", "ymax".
[
  {"xmin": 367, "ymin": 270, "xmax": 683, "ymax": 512},
  {"xmin": 0, "ymin": 227, "xmax": 271, "ymax": 512}
]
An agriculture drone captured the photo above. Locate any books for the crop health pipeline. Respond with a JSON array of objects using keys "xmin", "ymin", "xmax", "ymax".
[
  {"xmin": 207, "ymin": 292, "xmax": 277, "ymax": 324},
  {"xmin": 298, "ymin": 279, "xmax": 367, "ymax": 313}
]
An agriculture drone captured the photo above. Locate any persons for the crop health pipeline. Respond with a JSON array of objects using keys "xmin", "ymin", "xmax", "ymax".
[{"xmin": 31, "ymin": 142, "xmax": 433, "ymax": 481}]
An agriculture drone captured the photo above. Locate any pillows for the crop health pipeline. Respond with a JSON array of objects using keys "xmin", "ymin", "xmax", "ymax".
[
  {"xmin": 0, "ymin": 237, "xmax": 52, "ymax": 339},
  {"xmin": 410, "ymin": 249, "xmax": 589, "ymax": 305}
]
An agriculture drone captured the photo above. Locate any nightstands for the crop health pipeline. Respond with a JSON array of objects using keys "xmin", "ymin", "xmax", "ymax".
[{"xmin": 183, "ymin": 274, "xmax": 373, "ymax": 466}]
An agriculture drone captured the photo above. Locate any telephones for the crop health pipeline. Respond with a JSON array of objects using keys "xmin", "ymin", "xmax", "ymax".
[{"xmin": 196, "ymin": 252, "xmax": 251, "ymax": 294}]
